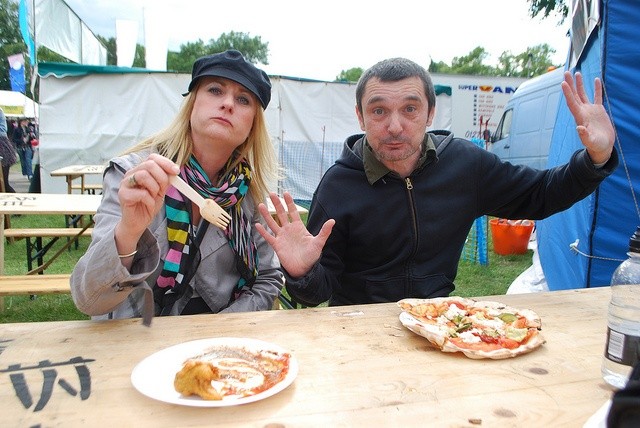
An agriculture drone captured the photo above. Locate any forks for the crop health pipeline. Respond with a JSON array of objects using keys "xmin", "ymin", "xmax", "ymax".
[{"xmin": 169, "ymin": 174, "xmax": 232, "ymax": 231}]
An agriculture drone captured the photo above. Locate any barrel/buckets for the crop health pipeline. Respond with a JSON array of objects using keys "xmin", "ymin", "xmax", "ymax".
[{"xmin": 489, "ymin": 218, "xmax": 535, "ymax": 256}]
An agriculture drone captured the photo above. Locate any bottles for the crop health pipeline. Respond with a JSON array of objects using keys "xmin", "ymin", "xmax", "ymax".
[{"xmin": 600, "ymin": 225, "xmax": 639, "ymax": 391}]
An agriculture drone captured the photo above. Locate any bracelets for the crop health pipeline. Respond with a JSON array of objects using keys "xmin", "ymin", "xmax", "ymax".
[{"xmin": 118, "ymin": 250, "xmax": 139, "ymax": 258}]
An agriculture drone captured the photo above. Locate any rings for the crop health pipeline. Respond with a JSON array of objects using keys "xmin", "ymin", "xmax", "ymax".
[{"xmin": 128, "ymin": 174, "xmax": 137, "ymax": 188}]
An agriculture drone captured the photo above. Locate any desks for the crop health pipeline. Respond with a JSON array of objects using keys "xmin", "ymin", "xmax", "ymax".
[
  {"xmin": 1, "ymin": 192, "xmax": 103, "ymax": 223},
  {"xmin": 51, "ymin": 164, "xmax": 108, "ymax": 229}
]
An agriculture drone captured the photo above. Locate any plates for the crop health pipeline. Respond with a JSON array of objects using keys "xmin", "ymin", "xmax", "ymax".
[
  {"xmin": 398, "ymin": 295, "xmax": 542, "ymax": 339},
  {"xmin": 131, "ymin": 336, "xmax": 299, "ymax": 408}
]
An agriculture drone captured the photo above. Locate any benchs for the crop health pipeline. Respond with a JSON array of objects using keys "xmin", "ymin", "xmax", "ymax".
[
  {"xmin": 4, "ymin": 228, "xmax": 94, "ymax": 301},
  {"xmin": 0, "ymin": 274, "xmax": 94, "ymax": 295},
  {"xmin": 70, "ymin": 183, "xmax": 104, "ymax": 195}
]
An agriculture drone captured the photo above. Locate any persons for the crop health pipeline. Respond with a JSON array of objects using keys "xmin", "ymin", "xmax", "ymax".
[
  {"xmin": 0, "ymin": 106, "xmax": 17, "ymax": 193},
  {"xmin": 13, "ymin": 116, "xmax": 34, "ymax": 180},
  {"xmin": 255, "ymin": 57, "xmax": 619, "ymax": 307},
  {"xmin": 70, "ymin": 49, "xmax": 286, "ymax": 322}
]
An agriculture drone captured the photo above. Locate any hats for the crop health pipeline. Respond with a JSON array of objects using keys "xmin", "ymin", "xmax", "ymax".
[{"xmin": 182, "ymin": 49, "xmax": 271, "ymax": 110}]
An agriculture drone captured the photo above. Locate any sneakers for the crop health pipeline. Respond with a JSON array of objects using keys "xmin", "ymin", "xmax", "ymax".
[{"xmin": 29, "ymin": 175, "xmax": 32, "ymax": 181}]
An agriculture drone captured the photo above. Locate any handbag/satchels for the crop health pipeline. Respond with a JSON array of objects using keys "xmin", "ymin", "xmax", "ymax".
[{"xmin": 0, "ymin": 132, "xmax": 17, "ymax": 167}]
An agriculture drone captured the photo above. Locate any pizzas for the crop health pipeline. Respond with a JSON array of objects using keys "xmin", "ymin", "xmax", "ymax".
[{"xmin": 396, "ymin": 295, "xmax": 546, "ymax": 359}]
